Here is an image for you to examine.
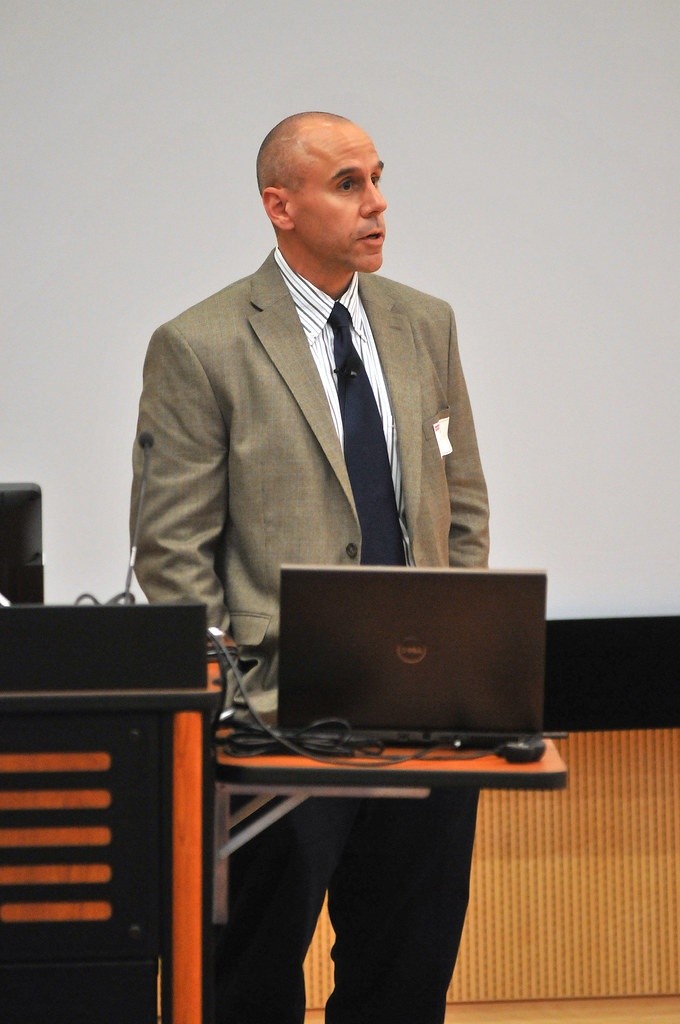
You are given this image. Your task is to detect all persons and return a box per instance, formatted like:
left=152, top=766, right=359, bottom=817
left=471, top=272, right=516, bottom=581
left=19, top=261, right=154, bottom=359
left=128, top=112, right=488, bottom=1024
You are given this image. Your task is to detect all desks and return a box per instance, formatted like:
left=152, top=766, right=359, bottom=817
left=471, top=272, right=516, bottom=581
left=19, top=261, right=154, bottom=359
left=211, top=715, right=568, bottom=926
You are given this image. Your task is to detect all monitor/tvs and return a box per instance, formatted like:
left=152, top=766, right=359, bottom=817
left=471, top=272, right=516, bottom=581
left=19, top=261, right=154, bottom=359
left=0, top=482, right=44, bottom=604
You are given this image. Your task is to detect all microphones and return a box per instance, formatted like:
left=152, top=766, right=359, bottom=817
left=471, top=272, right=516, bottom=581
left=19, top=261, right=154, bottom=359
left=125, top=432, right=154, bottom=606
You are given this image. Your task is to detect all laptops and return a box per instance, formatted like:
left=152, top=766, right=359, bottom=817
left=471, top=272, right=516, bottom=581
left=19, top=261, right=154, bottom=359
left=276, top=563, right=547, bottom=745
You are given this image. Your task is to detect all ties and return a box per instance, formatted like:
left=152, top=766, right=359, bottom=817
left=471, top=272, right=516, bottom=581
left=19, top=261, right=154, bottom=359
left=327, top=301, right=406, bottom=569
